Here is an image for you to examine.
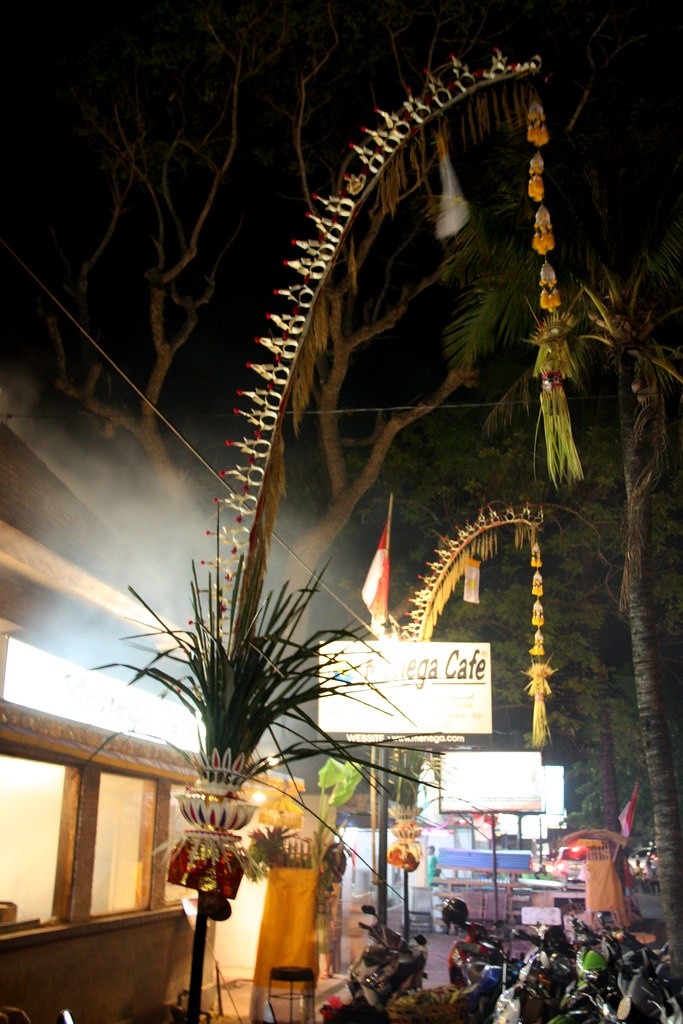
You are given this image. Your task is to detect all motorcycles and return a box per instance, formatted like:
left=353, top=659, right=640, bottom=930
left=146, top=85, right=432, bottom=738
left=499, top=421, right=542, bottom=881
left=344, top=897, right=683, bottom=1024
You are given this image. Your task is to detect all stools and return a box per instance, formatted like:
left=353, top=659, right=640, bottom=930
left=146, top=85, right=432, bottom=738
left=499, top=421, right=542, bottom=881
left=268, top=966, right=315, bottom=1000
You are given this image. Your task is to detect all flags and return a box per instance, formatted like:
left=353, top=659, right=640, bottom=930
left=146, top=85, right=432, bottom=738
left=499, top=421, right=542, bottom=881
left=618, top=783, right=637, bottom=848
left=361, top=523, right=389, bottom=618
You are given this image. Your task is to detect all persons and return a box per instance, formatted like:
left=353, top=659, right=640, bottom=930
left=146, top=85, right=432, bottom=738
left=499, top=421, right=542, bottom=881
left=315, top=886, right=334, bottom=980
left=427, top=846, right=437, bottom=888
left=521, top=866, right=553, bottom=880
left=624, top=853, right=661, bottom=897
